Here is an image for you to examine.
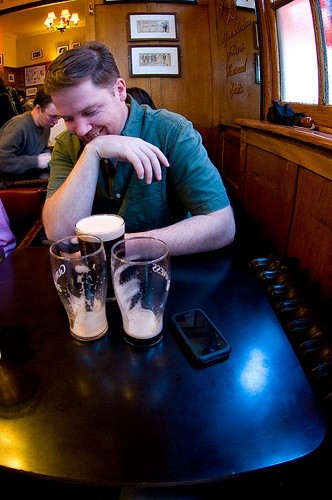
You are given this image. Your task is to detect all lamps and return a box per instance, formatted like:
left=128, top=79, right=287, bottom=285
left=44, top=7, right=80, bottom=35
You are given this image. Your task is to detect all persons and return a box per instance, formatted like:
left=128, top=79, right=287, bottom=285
left=0, top=199, right=17, bottom=258
left=43, top=42, right=236, bottom=262
left=126, top=86, right=157, bottom=110
left=0, top=78, right=60, bottom=173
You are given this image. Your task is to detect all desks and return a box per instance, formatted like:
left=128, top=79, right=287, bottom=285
left=0, top=238, right=327, bottom=488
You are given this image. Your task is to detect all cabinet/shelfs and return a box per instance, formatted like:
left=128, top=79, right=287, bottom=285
left=242, top=142, right=332, bottom=303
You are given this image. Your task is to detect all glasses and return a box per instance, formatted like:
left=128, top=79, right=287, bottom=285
left=41, top=106, right=61, bottom=121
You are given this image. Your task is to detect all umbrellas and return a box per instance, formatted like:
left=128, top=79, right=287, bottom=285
left=265, top=100, right=317, bottom=131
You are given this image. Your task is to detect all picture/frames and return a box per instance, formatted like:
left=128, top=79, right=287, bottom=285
left=128, top=44, right=181, bottom=78
left=26, top=87, right=37, bottom=97
left=24, top=64, right=46, bottom=87
left=31, top=49, right=42, bottom=59
left=56, top=45, right=68, bottom=54
left=125, top=11, right=180, bottom=42
left=8, top=74, right=15, bottom=82
left=0, top=54, right=3, bottom=65
left=72, top=42, right=81, bottom=48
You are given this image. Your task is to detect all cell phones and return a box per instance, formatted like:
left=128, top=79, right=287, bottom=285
left=42, top=239, right=80, bottom=253
left=170, top=308, right=232, bottom=366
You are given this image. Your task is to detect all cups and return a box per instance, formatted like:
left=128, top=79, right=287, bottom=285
left=49, top=233, right=109, bottom=342
left=75, top=213, right=129, bottom=302
left=110, top=237, right=171, bottom=349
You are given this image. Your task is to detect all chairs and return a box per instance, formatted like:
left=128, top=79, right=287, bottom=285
left=0, top=188, right=45, bottom=247
left=0, top=179, right=49, bottom=188
